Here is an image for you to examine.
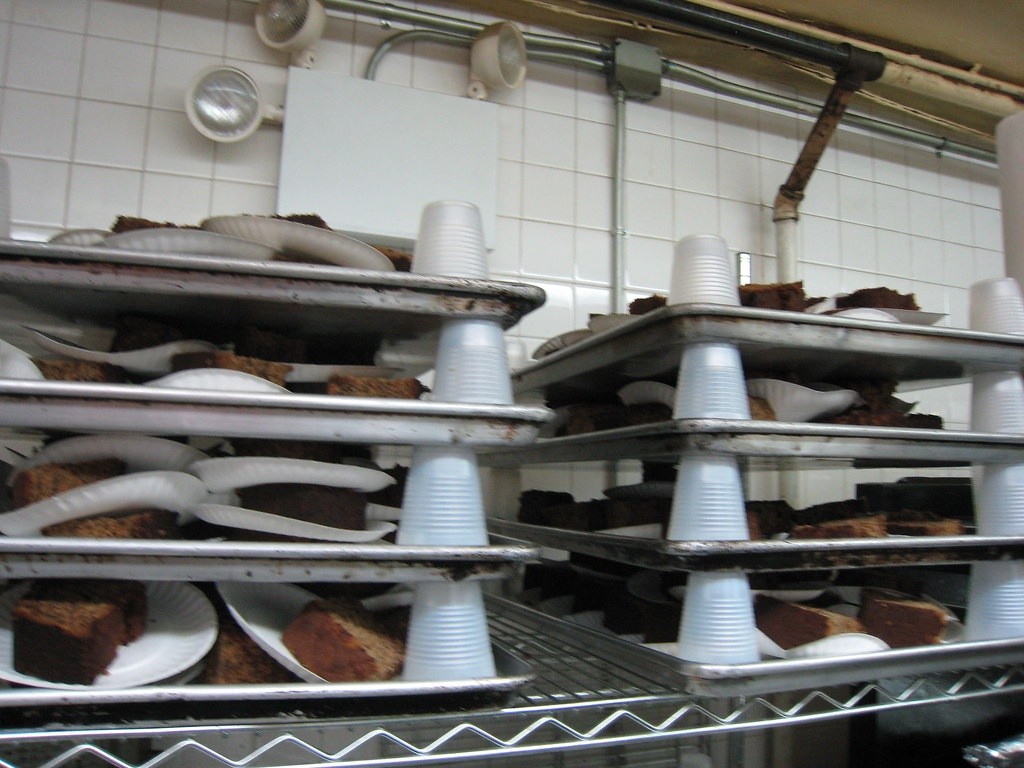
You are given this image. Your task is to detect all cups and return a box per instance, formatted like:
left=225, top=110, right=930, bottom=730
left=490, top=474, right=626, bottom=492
left=667, top=452, right=749, bottom=541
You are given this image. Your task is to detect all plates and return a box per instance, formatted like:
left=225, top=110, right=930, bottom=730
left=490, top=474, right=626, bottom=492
left=0, top=208, right=419, bottom=692
left=741, top=294, right=951, bottom=658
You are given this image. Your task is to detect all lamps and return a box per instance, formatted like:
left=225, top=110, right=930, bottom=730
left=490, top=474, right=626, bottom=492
left=252, top=0, right=326, bottom=68
left=467, top=21, right=529, bottom=98
left=183, top=64, right=286, bottom=144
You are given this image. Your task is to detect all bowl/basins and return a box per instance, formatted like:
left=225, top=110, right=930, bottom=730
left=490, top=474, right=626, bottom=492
left=965, top=562, right=1023, bottom=644
left=967, top=276, right=1023, bottom=337
left=678, top=571, right=761, bottom=665
left=971, top=370, right=1024, bottom=436
left=667, top=236, right=739, bottom=308
left=431, top=319, right=513, bottom=404
left=412, top=201, right=490, bottom=280
left=671, top=340, right=749, bottom=423
left=398, top=581, right=498, bottom=681
left=972, top=463, right=1023, bottom=537
left=394, top=447, right=489, bottom=549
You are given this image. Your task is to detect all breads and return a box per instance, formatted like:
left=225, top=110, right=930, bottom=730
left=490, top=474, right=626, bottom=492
left=6, top=211, right=424, bottom=680
left=514, top=280, right=967, bottom=654
